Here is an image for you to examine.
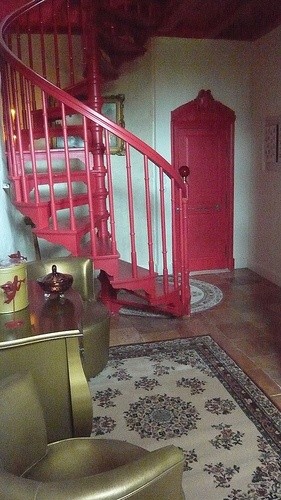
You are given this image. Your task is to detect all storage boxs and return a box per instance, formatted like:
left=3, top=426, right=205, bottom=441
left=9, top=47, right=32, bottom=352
left=0, top=258, right=30, bottom=313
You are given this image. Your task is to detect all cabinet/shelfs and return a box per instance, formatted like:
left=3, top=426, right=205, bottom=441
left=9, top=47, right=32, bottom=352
left=0, top=278, right=93, bottom=444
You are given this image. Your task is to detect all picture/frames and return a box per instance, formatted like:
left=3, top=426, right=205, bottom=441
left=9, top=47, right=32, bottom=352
left=262, top=116, right=281, bottom=172
left=47, top=93, right=126, bottom=156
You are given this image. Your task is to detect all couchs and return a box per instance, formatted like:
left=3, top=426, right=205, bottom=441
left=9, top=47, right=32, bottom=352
left=1, top=369, right=187, bottom=500
left=27, top=256, right=111, bottom=381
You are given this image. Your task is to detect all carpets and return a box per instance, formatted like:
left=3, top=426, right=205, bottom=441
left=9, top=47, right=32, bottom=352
left=114, top=274, right=224, bottom=318
left=87, top=334, right=281, bottom=500
